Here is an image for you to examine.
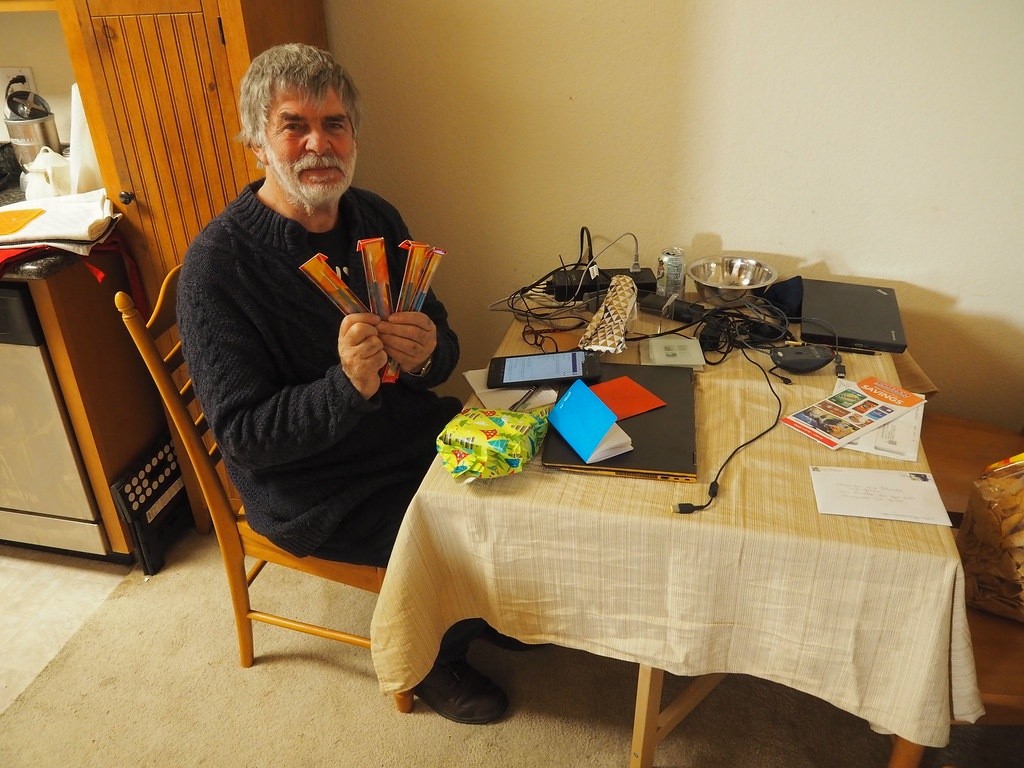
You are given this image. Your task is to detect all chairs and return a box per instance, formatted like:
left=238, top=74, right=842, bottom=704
left=116, top=265, right=415, bottom=713
left=885, top=610, right=1024, bottom=768
left=920, top=413, right=1024, bottom=523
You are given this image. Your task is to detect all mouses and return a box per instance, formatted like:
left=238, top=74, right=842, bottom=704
left=771, top=346, right=836, bottom=373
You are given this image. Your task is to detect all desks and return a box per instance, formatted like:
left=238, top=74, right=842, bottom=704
left=368, top=290, right=984, bottom=768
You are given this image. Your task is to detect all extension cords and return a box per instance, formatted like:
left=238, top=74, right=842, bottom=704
left=544, top=268, right=657, bottom=302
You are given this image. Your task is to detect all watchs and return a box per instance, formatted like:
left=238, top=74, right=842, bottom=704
left=408, top=355, right=434, bottom=377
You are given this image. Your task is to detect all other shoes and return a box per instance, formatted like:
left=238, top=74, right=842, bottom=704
left=475, top=622, right=541, bottom=651
left=412, top=648, right=509, bottom=724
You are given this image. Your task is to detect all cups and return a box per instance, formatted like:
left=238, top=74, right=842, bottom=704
left=5, top=114, right=59, bottom=171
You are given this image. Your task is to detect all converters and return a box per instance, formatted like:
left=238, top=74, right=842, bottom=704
left=583, top=288, right=704, bottom=323
left=699, top=316, right=729, bottom=350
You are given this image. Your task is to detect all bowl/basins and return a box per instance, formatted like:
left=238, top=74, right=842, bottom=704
left=686, top=256, right=777, bottom=305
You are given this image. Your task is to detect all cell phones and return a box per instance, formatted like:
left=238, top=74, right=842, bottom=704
left=487, top=351, right=601, bottom=388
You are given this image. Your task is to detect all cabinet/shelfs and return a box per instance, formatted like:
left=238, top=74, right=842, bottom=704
left=76, top=0, right=328, bottom=535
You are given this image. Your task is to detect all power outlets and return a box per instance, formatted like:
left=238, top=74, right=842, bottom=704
left=0, top=66, right=36, bottom=100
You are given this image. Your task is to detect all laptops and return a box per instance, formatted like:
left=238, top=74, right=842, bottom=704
left=542, top=363, right=697, bottom=482
left=801, top=279, right=907, bottom=353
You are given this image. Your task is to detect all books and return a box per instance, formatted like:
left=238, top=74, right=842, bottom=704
left=462, top=369, right=558, bottom=411
left=548, top=379, right=633, bottom=463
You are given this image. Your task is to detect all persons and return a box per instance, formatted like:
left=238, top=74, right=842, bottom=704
left=177, top=44, right=539, bottom=724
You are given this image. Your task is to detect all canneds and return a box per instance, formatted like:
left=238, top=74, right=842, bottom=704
left=656, top=247, right=686, bottom=299
